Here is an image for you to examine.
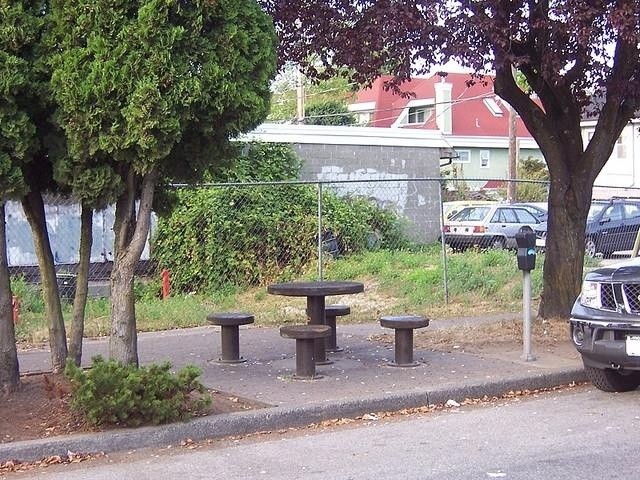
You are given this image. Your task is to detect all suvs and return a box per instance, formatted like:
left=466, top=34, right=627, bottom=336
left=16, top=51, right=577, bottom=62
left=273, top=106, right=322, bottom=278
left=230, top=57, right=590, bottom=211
left=568, top=255, right=640, bottom=391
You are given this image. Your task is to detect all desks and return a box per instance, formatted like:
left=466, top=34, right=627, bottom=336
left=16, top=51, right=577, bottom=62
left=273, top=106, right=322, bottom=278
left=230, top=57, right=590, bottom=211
left=268, top=281, right=364, bottom=365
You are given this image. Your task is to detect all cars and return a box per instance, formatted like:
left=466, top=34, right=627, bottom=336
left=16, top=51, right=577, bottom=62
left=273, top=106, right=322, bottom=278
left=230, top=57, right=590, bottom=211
left=534, top=196, right=640, bottom=258
left=442, top=201, right=548, bottom=249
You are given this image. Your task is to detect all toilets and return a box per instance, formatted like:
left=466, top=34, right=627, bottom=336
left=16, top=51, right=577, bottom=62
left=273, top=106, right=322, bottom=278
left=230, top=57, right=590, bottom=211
left=56, top=273, right=76, bottom=305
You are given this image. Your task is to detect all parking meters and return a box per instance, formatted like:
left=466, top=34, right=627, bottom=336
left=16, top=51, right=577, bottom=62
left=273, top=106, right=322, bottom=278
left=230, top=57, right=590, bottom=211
left=512, top=224, right=542, bottom=363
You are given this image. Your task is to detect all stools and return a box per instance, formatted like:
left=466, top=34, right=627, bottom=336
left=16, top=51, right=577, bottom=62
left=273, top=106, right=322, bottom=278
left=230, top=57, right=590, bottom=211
left=206, top=303, right=429, bottom=379
